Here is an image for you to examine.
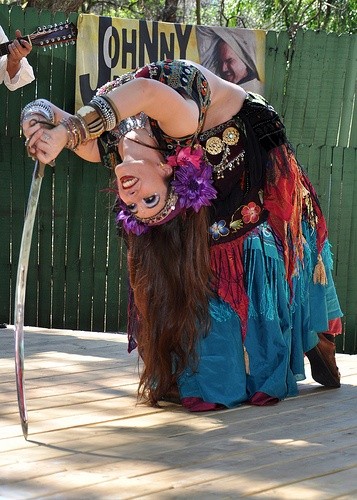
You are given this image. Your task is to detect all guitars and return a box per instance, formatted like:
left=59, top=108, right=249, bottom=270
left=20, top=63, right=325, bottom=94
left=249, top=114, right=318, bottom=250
left=1, top=17, right=79, bottom=60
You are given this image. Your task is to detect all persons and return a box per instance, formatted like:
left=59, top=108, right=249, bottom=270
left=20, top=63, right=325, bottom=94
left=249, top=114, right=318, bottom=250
left=21, top=59, right=343, bottom=412
left=0, top=26, right=35, bottom=91
left=196, top=26, right=263, bottom=96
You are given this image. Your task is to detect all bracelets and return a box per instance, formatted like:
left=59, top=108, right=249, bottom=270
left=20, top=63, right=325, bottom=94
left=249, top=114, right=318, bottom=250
left=59, top=95, right=122, bottom=151
left=20, top=100, right=55, bottom=125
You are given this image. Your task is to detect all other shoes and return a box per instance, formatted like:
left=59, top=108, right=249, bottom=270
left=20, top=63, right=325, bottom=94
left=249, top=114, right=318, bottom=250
left=304, top=332, right=341, bottom=388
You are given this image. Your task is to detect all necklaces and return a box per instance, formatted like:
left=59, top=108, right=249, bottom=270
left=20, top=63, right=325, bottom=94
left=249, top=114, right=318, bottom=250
left=121, top=134, right=158, bottom=150
